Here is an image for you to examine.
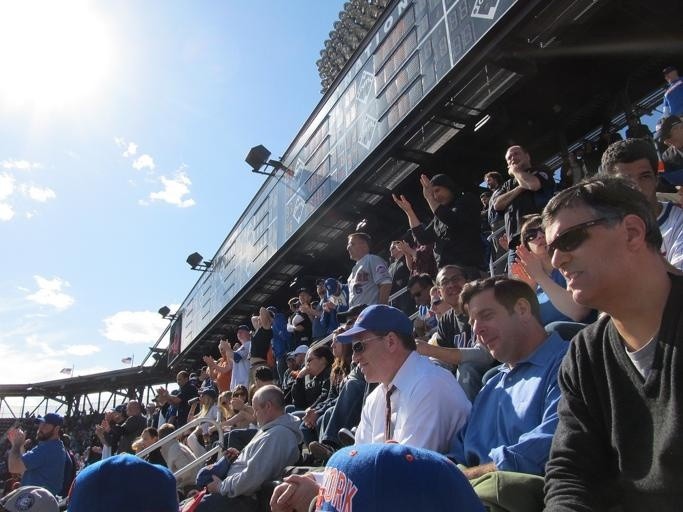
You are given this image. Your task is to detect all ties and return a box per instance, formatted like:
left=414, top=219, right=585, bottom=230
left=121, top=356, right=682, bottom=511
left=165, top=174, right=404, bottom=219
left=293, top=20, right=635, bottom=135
left=383, top=385, right=398, bottom=440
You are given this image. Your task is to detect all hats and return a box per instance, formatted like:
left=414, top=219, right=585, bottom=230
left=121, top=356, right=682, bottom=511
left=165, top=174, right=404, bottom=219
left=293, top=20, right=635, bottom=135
left=37, top=412, right=64, bottom=426
left=66, top=451, right=180, bottom=512
left=296, top=287, right=314, bottom=298
left=111, top=404, right=124, bottom=412
left=197, top=389, right=217, bottom=399
left=316, top=439, right=487, bottom=512
left=335, top=303, right=414, bottom=344
left=324, top=278, right=337, bottom=296
left=234, top=324, right=251, bottom=333
left=0, top=485, right=60, bottom=512
left=195, top=455, right=229, bottom=491
left=429, top=172, right=457, bottom=193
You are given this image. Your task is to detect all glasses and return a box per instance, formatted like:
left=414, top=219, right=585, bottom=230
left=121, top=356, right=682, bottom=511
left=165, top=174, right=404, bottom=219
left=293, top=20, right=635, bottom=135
left=351, top=334, right=383, bottom=355
left=232, top=392, right=242, bottom=397
left=523, top=226, right=545, bottom=242
left=290, top=344, right=310, bottom=356
left=548, top=215, right=622, bottom=259
left=414, top=287, right=426, bottom=298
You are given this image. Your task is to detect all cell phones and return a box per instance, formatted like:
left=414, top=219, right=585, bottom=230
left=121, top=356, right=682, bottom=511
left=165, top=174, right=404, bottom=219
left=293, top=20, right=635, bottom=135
left=219, top=442, right=232, bottom=455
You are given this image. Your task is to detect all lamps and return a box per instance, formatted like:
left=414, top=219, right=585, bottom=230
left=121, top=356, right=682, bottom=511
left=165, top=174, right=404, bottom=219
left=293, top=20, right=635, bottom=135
left=147, top=345, right=168, bottom=361
left=185, top=250, right=215, bottom=273
left=157, top=305, right=179, bottom=322
left=244, top=142, right=294, bottom=181
